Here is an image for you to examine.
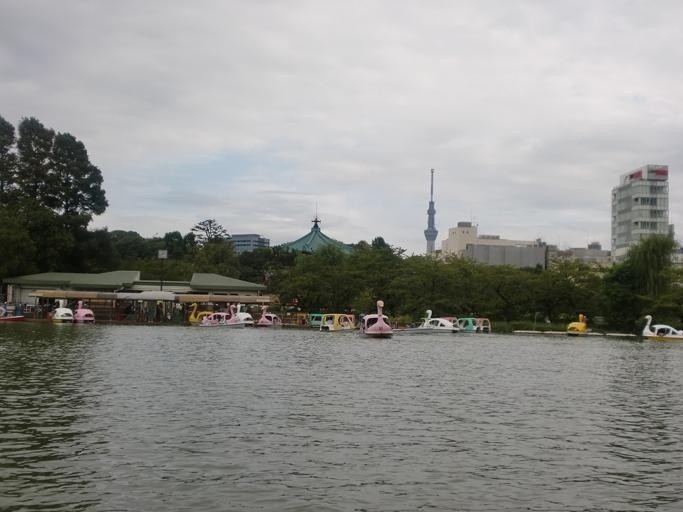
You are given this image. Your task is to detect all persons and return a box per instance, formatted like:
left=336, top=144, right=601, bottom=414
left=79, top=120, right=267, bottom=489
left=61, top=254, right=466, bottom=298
left=115, top=300, right=265, bottom=323
left=358, top=313, right=365, bottom=326
left=407, top=321, right=417, bottom=328
left=0, top=299, right=89, bottom=318
left=544, top=315, right=550, bottom=326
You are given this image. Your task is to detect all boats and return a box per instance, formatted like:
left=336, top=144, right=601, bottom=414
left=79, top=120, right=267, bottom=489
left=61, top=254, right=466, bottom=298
left=566, top=314, right=586, bottom=334
left=641, top=315, right=683, bottom=340
left=185, top=294, right=356, bottom=332
left=73, top=301, right=97, bottom=323
left=1, top=316, right=24, bottom=322
left=359, top=300, right=393, bottom=336
left=417, top=310, right=457, bottom=330
left=51, top=299, right=74, bottom=323
left=454, top=317, right=492, bottom=333
